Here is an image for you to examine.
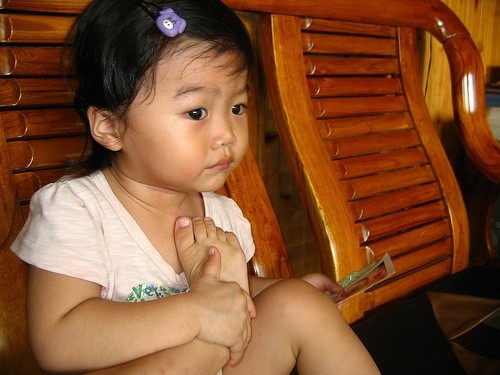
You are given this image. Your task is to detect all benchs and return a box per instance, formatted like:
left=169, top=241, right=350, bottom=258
left=0, top=0, right=500, bottom=375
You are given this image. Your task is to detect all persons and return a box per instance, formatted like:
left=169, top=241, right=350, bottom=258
left=10, top=1, right=381, bottom=375
